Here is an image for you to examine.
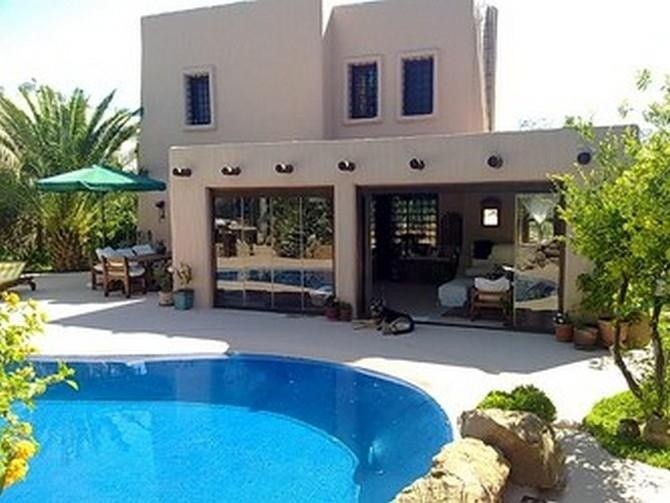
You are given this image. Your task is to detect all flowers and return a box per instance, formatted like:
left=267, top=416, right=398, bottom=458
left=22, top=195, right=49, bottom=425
left=551, top=310, right=569, bottom=325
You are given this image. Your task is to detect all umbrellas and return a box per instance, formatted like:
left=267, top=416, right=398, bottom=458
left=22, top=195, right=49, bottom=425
left=32, top=162, right=167, bottom=247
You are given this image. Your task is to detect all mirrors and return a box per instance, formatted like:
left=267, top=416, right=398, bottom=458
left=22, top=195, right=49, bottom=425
left=480, top=206, right=501, bottom=227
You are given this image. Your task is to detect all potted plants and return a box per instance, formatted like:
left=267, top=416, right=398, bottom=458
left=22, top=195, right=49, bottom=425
left=151, top=260, right=195, bottom=311
left=571, top=302, right=619, bottom=349
left=319, top=295, right=352, bottom=321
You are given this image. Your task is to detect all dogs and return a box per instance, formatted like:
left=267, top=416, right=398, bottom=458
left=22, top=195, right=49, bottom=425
left=368, top=295, right=415, bottom=335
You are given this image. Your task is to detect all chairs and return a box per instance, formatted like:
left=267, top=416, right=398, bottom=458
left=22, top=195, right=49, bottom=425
left=469, top=284, right=514, bottom=321
left=466, top=239, right=495, bottom=275
left=87, top=239, right=172, bottom=299
left=0, top=261, right=36, bottom=300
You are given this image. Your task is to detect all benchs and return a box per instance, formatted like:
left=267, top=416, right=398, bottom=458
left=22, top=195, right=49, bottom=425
left=436, top=275, right=474, bottom=317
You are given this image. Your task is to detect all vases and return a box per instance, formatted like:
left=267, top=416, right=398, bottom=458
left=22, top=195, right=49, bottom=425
left=555, top=324, right=573, bottom=342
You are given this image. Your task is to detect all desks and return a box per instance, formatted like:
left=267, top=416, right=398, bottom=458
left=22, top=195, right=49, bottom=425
left=399, top=255, right=456, bottom=282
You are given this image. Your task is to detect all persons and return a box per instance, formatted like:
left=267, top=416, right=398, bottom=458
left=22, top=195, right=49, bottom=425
left=521, top=247, right=551, bottom=270
